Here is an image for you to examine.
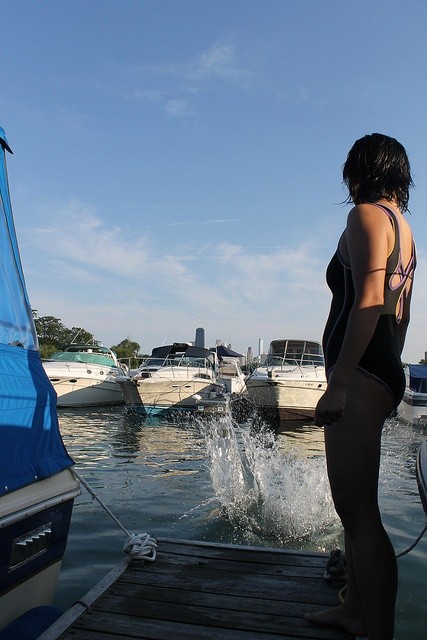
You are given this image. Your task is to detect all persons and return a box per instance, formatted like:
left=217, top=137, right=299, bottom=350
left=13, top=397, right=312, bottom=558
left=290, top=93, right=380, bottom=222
left=304, top=132, right=417, bottom=640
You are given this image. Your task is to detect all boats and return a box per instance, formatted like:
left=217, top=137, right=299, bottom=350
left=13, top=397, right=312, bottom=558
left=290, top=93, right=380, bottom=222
left=0, top=131, right=81, bottom=639
left=398, top=364, right=427, bottom=428
left=42, top=346, right=131, bottom=408
left=114, top=343, right=246, bottom=415
left=244, top=338, right=327, bottom=422
